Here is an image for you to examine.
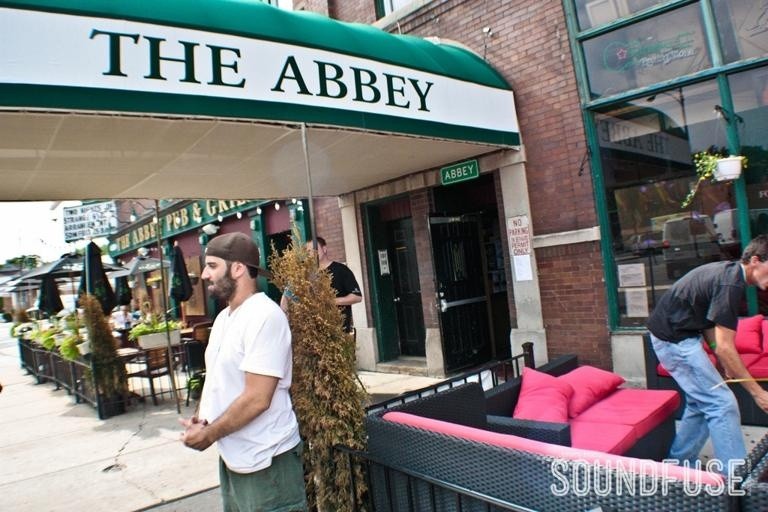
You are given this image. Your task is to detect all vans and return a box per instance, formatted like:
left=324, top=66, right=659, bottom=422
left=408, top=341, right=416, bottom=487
left=632, top=205, right=767, bottom=280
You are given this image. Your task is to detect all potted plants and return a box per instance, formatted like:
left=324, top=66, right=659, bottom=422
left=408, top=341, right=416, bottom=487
left=681, top=150, right=746, bottom=208
left=36, top=326, right=92, bottom=364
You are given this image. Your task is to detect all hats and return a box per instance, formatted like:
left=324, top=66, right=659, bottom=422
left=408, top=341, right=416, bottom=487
left=205, top=232, right=272, bottom=278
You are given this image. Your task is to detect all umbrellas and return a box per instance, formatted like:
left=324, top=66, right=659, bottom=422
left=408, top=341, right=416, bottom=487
left=0, top=236, right=195, bottom=323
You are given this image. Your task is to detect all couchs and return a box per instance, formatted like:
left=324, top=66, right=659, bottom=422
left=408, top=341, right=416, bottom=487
left=348, top=314, right=767, bottom=512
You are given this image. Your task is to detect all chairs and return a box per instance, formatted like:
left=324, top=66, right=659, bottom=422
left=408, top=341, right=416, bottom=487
left=114, top=320, right=212, bottom=408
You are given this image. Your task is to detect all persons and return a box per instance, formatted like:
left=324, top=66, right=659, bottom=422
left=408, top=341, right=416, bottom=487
left=128, top=301, right=155, bottom=328
left=109, top=303, right=132, bottom=338
left=644, top=233, right=768, bottom=480
left=278, top=261, right=309, bottom=314
left=177, top=231, right=311, bottom=512
left=304, top=235, right=364, bottom=338
